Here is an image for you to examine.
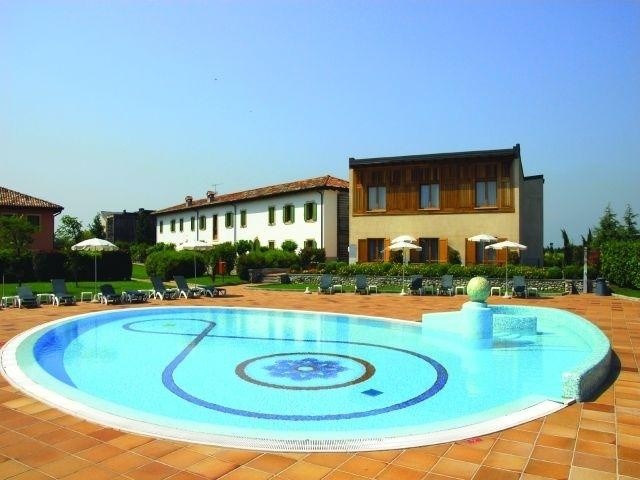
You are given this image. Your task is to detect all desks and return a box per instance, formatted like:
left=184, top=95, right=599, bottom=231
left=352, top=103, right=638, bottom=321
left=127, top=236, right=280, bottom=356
left=1, top=296, right=15, bottom=308
left=368, top=285, right=378, bottom=293
left=490, top=286, right=503, bottom=296
left=455, top=286, right=466, bottom=295
left=81, top=291, right=92, bottom=303
left=527, top=287, right=538, bottom=297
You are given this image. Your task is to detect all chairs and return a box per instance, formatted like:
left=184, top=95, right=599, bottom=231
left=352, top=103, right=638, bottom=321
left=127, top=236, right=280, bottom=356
left=16, top=279, right=74, bottom=307
left=512, top=275, right=528, bottom=298
left=99, top=272, right=226, bottom=305
left=317, top=273, right=370, bottom=295
left=407, top=274, right=455, bottom=296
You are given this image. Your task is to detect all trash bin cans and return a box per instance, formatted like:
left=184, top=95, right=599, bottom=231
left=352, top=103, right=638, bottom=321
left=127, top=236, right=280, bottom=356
left=248, top=272, right=255, bottom=282
left=596, top=278, right=606, bottom=295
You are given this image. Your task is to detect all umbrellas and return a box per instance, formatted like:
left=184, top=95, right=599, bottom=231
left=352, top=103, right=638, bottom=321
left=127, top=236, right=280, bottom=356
left=389, top=242, right=423, bottom=289
left=391, top=235, right=417, bottom=262
left=468, top=233, right=498, bottom=264
left=484, top=241, right=527, bottom=291
left=71, top=238, right=119, bottom=295
